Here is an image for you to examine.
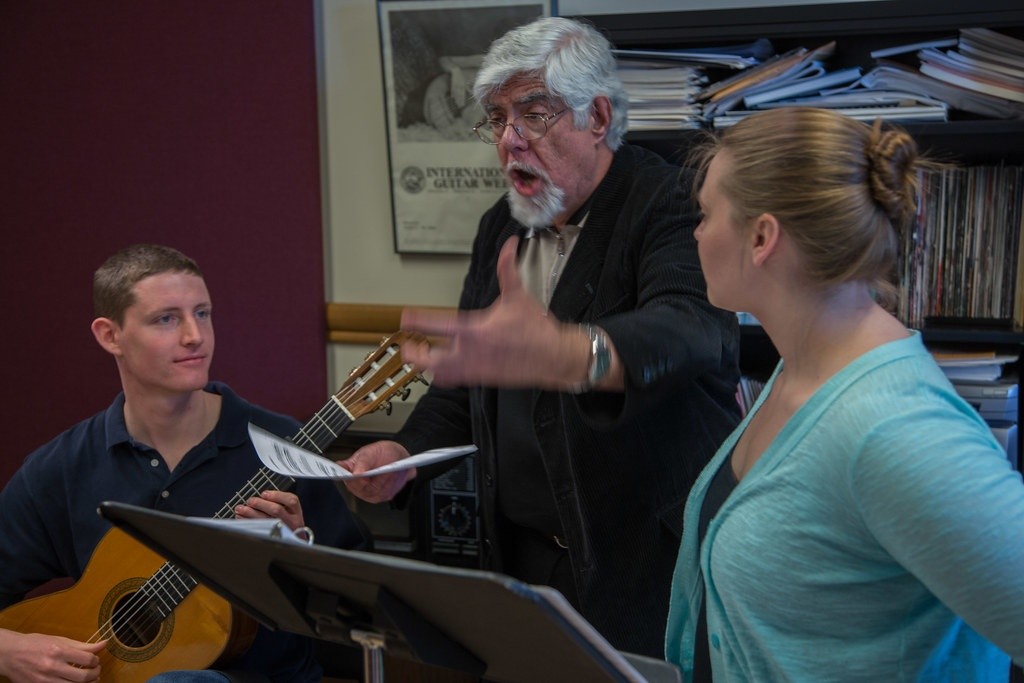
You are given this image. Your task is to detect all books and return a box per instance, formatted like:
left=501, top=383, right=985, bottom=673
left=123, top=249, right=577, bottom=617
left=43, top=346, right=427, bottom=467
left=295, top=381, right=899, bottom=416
left=608, top=25, right=1024, bottom=131
left=892, top=159, right=1024, bottom=331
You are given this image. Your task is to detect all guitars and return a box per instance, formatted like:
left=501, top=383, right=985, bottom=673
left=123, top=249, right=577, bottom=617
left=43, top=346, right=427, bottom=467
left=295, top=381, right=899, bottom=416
left=0, top=326, right=435, bottom=683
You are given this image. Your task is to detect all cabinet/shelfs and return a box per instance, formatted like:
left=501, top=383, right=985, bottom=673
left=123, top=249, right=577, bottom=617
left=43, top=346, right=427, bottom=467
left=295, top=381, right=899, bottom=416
left=552, top=0, right=1024, bottom=477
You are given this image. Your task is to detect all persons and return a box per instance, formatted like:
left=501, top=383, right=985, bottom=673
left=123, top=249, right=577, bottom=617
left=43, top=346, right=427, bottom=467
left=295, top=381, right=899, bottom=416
left=336, top=14, right=745, bottom=658
left=662, top=106, right=1024, bottom=683
left=0, top=241, right=373, bottom=683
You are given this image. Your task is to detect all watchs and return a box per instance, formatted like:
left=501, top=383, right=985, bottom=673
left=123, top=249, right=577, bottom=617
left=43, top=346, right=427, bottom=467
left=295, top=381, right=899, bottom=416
left=570, top=325, right=612, bottom=395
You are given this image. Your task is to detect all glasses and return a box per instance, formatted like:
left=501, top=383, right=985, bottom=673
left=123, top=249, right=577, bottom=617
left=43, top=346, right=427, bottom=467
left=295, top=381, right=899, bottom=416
left=473, top=104, right=572, bottom=145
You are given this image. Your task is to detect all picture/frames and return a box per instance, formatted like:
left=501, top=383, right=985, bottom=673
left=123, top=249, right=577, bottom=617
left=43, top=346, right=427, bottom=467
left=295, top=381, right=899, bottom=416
left=376, top=0, right=556, bottom=255
